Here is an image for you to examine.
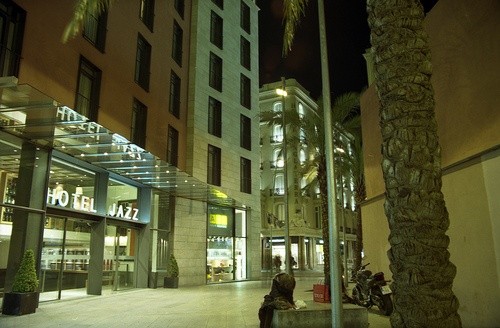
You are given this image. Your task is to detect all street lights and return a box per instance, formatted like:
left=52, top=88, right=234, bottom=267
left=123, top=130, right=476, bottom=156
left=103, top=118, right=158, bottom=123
left=275, top=75, right=293, bottom=277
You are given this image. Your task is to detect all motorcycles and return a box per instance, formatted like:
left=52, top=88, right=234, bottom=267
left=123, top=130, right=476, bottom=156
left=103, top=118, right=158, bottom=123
left=351, top=262, right=395, bottom=316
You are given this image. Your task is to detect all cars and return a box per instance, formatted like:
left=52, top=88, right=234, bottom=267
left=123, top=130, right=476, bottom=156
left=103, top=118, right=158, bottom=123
left=42, top=247, right=90, bottom=267
